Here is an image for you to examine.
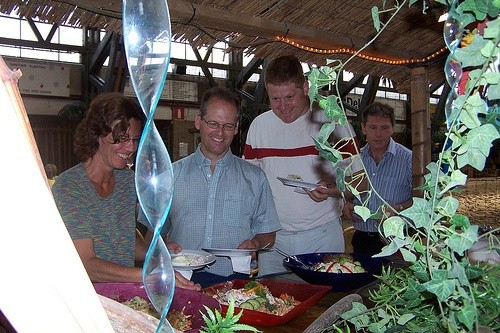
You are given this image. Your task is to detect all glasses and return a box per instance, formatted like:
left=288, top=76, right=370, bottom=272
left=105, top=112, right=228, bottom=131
left=108, top=135, right=141, bottom=144
left=202, top=117, right=237, bottom=131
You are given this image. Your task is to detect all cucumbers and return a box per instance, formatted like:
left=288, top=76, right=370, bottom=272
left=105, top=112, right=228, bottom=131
left=353, top=261, right=364, bottom=273
left=239, top=298, right=268, bottom=310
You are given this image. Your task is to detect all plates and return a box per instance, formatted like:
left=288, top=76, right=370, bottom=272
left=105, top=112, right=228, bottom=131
left=201, top=248, right=258, bottom=256
left=276, top=176, right=322, bottom=190
left=195, top=278, right=332, bottom=327
left=168, top=249, right=216, bottom=271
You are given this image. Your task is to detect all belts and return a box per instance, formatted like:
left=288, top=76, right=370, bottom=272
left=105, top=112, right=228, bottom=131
left=357, top=230, right=385, bottom=236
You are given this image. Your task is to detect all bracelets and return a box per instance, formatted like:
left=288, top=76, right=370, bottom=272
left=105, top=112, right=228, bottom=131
left=399, top=204, right=403, bottom=210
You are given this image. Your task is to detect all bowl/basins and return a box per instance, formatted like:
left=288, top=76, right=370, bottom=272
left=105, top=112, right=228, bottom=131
left=282, top=252, right=389, bottom=292
left=92, top=282, right=222, bottom=333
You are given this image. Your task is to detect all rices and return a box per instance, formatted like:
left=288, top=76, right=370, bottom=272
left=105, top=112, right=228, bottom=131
left=171, top=255, right=204, bottom=266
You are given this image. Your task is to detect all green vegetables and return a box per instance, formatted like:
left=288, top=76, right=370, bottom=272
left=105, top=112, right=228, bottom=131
left=331, top=254, right=350, bottom=265
left=244, top=282, right=267, bottom=298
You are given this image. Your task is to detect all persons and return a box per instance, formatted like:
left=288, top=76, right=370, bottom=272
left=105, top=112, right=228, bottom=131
left=51, top=91, right=202, bottom=292
left=137, top=86, right=282, bottom=289
left=343, top=102, right=413, bottom=252
left=241, top=54, right=366, bottom=277
